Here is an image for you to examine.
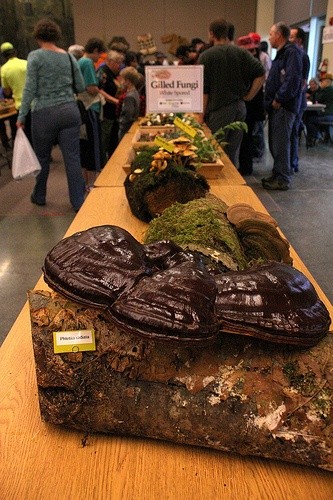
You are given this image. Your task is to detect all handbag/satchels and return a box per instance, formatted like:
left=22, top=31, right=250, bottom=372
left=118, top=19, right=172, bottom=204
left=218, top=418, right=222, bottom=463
left=77, top=99, right=88, bottom=125
left=11, top=126, right=42, bottom=182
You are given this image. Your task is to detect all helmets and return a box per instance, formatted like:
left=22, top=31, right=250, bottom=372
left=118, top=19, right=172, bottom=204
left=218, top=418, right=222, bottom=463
left=0, top=42, right=14, bottom=52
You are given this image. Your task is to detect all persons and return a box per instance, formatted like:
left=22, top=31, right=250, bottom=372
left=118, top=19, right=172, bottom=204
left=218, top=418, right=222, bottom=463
left=175, top=17, right=310, bottom=190
left=0, top=36, right=172, bottom=192
left=302, top=74, right=333, bottom=148
left=16, top=17, right=87, bottom=211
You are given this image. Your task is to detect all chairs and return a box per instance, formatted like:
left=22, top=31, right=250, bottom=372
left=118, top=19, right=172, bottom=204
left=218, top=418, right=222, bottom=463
left=319, top=118, right=333, bottom=144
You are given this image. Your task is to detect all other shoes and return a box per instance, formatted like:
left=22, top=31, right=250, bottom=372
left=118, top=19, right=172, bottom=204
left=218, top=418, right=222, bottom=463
left=260, top=171, right=290, bottom=191
left=31, top=193, right=46, bottom=206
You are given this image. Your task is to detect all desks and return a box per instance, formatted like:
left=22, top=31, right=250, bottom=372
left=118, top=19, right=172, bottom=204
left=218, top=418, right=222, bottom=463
left=94, top=125, right=249, bottom=184
left=0, top=186, right=333, bottom=499
left=301, top=101, right=327, bottom=147
left=0, top=110, right=17, bottom=176
left=128, top=115, right=212, bottom=132
